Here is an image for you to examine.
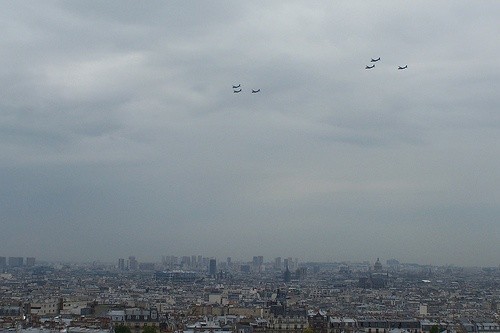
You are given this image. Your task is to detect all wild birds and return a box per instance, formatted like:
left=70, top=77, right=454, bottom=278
left=365, top=64, right=375, bottom=69
left=233, top=89, right=242, bottom=93
left=398, top=64, right=408, bottom=69
left=371, top=58, right=380, bottom=62
left=251, top=89, right=260, bottom=93
left=232, top=84, right=240, bottom=88
